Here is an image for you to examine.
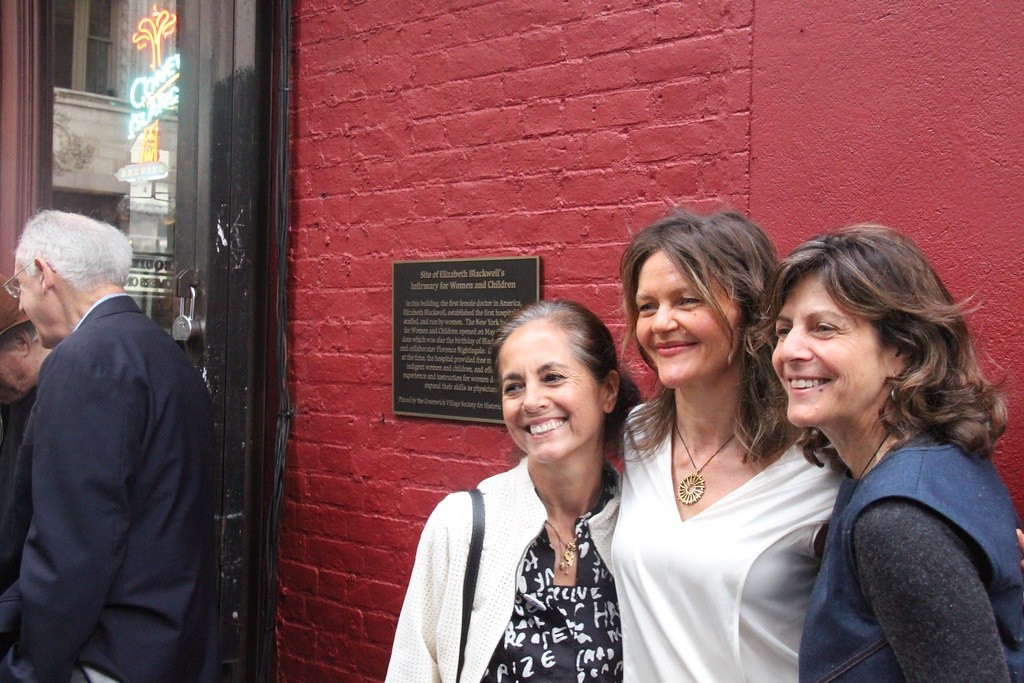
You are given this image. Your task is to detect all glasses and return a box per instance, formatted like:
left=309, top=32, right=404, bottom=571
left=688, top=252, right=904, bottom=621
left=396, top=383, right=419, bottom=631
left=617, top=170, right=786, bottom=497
left=2, top=258, right=56, bottom=298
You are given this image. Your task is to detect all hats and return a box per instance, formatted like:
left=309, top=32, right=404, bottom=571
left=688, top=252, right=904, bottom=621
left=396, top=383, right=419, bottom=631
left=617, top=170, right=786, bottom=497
left=0, top=273, right=31, bottom=334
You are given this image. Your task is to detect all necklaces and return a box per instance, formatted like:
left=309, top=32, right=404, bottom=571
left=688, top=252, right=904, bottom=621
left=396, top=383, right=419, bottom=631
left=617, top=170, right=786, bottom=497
left=547, top=521, right=577, bottom=575
left=846, top=428, right=891, bottom=500
left=676, top=417, right=748, bottom=504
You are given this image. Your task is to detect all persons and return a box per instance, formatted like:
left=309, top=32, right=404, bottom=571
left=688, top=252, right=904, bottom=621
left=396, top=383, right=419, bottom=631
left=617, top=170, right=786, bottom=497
left=384, top=301, right=642, bottom=683
left=612, top=212, right=1024, bottom=683
left=749, top=222, right=1024, bottom=683
left=0, top=210, right=217, bottom=683
